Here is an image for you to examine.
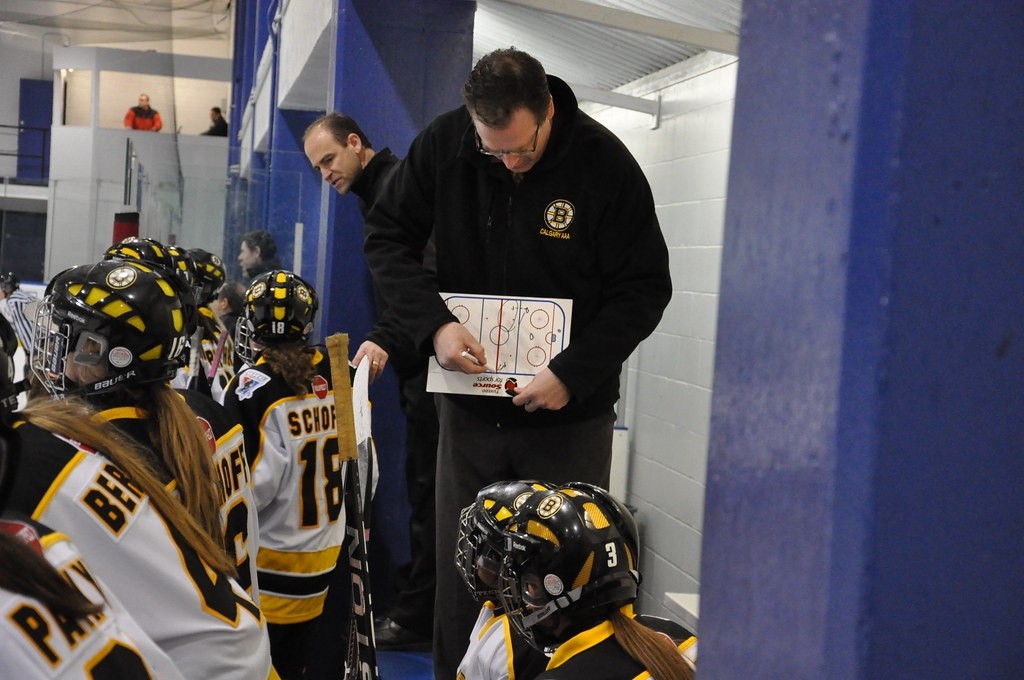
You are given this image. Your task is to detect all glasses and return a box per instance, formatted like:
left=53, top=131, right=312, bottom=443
left=471, top=124, right=540, bottom=158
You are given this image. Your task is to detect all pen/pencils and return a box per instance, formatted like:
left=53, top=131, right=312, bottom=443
left=461, top=351, right=496, bottom=372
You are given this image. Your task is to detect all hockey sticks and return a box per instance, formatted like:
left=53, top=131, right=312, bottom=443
left=322, top=327, right=381, bottom=680
left=205, top=330, right=230, bottom=394
left=186, top=325, right=205, bottom=390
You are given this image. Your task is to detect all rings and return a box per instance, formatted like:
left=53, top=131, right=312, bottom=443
left=373, top=361, right=378, bottom=365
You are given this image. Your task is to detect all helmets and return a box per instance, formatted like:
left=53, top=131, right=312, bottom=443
left=0, top=272, right=20, bottom=294
left=499, top=480, right=640, bottom=657
left=27, top=235, right=226, bottom=400
left=233, top=270, right=313, bottom=368
left=455, top=478, right=560, bottom=599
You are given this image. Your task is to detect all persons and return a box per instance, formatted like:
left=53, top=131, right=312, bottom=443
left=201, top=107, right=227, bottom=136
left=304, top=111, right=440, bottom=652
left=123, top=95, right=162, bottom=132
left=0, top=230, right=380, bottom=680
left=358, top=48, right=673, bottom=680
left=456, top=479, right=698, bottom=680
